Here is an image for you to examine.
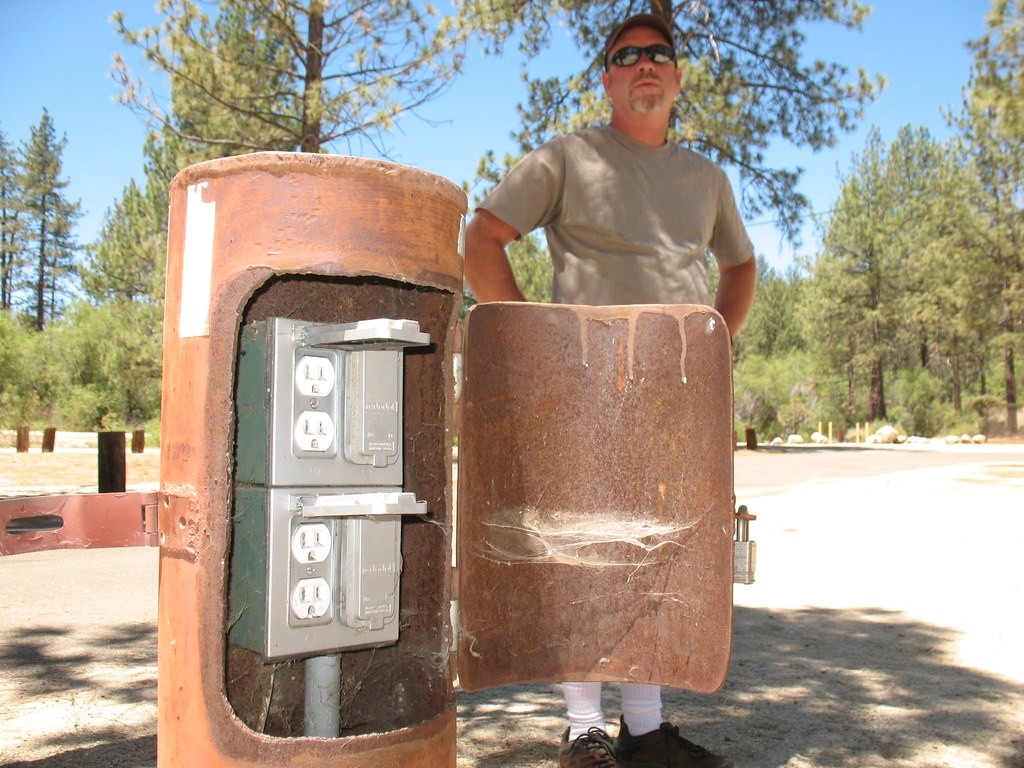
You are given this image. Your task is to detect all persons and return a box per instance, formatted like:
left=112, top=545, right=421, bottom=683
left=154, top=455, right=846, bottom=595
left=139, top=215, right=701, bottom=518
left=462, top=12, right=758, bottom=768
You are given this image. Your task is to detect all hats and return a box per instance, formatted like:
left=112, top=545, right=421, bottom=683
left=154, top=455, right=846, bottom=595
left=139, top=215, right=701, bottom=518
left=603, top=14, right=678, bottom=71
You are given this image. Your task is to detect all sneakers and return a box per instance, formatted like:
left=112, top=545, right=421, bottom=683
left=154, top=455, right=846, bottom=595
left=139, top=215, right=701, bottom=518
left=616, top=713, right=726, bottom=768
left=558, top=726, right=619, bottom=768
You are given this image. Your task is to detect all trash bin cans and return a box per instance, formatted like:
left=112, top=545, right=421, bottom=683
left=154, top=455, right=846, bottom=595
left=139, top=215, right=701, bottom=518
left=99, top=432, right=127, bottom=492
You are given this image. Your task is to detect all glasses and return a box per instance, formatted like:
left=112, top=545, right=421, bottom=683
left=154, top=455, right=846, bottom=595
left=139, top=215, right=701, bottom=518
left=605, top=43, right=675, bottom=71
